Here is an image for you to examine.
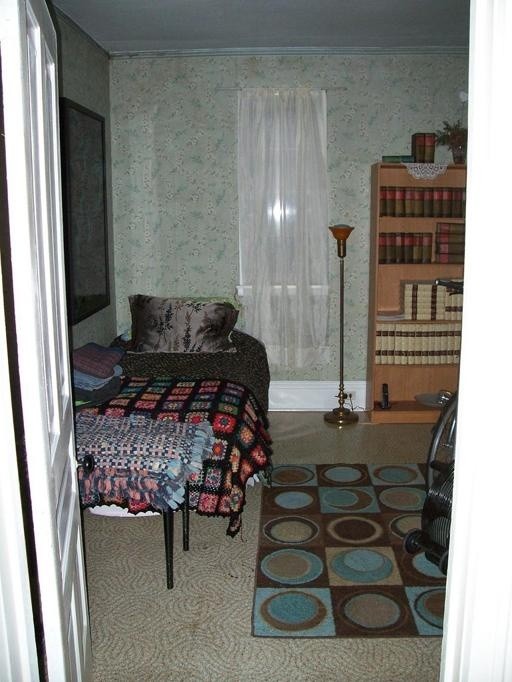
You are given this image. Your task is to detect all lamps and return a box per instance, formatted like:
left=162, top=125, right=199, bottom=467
left=323, top=224, right=358, bottom=426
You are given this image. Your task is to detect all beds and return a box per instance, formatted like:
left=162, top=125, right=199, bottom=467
left=73, top=327, right=272, bottom=541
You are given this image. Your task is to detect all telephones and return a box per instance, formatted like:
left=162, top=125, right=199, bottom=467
left=380, top=384, right=391, bottom=409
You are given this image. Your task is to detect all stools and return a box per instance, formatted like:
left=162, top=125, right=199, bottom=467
left=71, top=414, right=212, bottom=590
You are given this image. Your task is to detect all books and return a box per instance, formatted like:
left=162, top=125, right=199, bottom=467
left=374, top=186, right=466, bottom=366
left=381, top=383, right=389, bottom=409
left=381, top=133, right=436, bottom=164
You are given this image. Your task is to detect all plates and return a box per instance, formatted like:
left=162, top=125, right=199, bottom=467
left=415, top=394, right=444, bottom=407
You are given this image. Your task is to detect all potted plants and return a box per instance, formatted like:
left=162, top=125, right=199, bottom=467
left=434, top=119, right=468, bottom=163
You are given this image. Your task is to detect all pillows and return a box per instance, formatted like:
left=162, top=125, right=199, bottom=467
left=127, top=294, right=238, bottom=355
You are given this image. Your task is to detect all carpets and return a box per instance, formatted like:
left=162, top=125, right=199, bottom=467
left=250, top=462, right=450, bottom=637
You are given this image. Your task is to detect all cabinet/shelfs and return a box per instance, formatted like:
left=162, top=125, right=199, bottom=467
left=366, top=164, right=467, bottom=425
